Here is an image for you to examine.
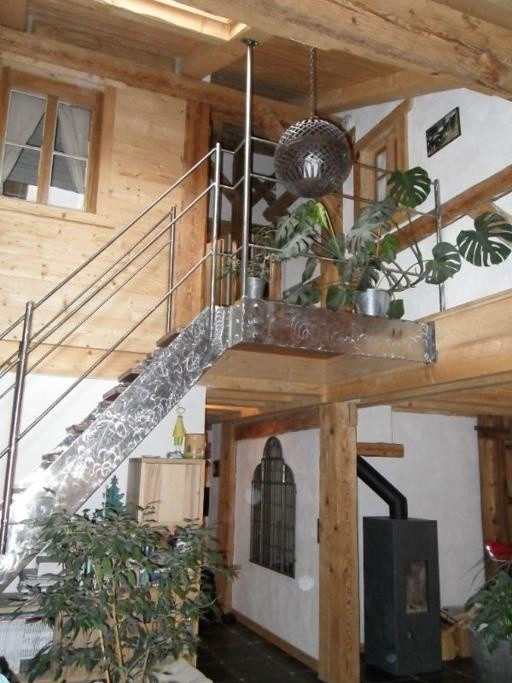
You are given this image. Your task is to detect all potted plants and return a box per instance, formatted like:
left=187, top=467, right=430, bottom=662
left=458, top=549, right=511, bottom=682
left=273, top=168, right=512, bottom=318
left=211, top=222, right=274, bottom=299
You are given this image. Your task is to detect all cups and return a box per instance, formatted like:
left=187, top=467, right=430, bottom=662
left=184, top=434, right=206, bottom=460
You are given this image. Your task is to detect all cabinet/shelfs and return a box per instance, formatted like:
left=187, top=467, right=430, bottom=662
left=128, top=458, right=206, bottom=669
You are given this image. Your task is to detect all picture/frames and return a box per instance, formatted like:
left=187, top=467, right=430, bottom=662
left=426, top=107, right=460, bottom=157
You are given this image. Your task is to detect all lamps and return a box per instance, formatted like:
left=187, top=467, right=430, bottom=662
left=272, top=47, right=353, bottom=199
